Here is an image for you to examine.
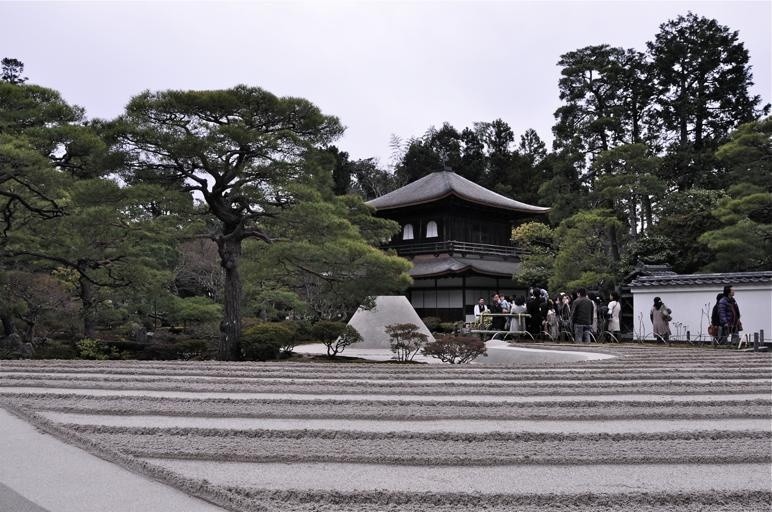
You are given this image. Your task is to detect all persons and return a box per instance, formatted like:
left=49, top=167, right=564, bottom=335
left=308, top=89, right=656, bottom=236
left=650, top=297, right=672, bottom=344
left=474, top=283, right=621, bottom=343
left=711, top=285, right=740, bottom=345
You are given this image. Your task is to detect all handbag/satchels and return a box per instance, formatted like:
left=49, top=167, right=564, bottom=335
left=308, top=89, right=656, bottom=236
left=707, top=325, right=720, bottom=337
left=660, top=305, right=672, bottom=321
